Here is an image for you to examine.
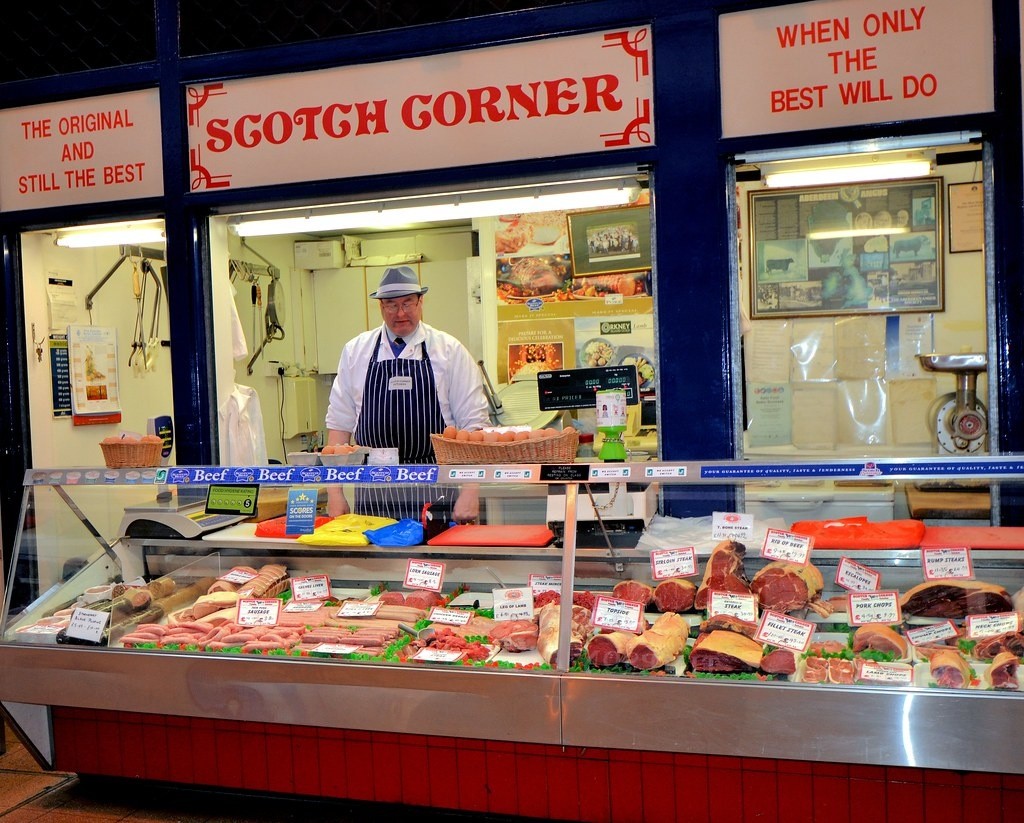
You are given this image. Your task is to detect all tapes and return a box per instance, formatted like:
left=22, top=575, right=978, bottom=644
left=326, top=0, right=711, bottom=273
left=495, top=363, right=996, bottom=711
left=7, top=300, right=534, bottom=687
left=422, top=503, right=432, bottom=531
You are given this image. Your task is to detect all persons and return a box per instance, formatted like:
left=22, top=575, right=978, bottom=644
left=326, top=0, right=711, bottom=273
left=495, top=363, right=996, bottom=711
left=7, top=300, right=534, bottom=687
left=324, top=266, right=492, bottom=524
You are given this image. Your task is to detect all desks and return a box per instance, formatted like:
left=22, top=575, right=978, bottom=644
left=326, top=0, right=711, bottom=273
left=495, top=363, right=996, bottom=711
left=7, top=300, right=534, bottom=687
left=903, top=483, right=993, bottom=527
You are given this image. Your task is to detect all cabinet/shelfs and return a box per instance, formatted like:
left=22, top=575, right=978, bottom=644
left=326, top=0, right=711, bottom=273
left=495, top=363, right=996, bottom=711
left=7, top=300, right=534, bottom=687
left=313, top=255, right=483, bottom=372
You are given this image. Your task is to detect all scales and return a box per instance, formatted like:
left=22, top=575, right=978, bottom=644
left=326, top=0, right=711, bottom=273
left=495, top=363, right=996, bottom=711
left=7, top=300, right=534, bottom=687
left=537, top=364, right=659, bottom=537
left=116, top=496, right=259, bottom=540
left=912, top=352, right=994, bottom=492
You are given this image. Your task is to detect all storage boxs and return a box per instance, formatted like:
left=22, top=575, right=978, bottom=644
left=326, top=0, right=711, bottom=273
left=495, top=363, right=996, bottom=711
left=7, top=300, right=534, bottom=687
left=286, top=453, right=320, bottom=466
left=416, top=231, right=479, bottom=259
left=320, top=446, right=370, bottom=468
left=360, top=236, right=415, bottom=255
left=294, top=239, right=345, bottom=271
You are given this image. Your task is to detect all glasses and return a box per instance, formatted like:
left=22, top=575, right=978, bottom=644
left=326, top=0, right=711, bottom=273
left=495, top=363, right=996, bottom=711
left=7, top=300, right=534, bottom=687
left=381, top=297, right=419, bottom=312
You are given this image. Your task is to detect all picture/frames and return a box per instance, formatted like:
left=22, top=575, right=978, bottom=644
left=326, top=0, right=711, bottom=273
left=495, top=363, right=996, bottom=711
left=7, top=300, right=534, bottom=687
left=564, top=204, right=656, bottom=276
left=746, top=173, right=948, bottom=321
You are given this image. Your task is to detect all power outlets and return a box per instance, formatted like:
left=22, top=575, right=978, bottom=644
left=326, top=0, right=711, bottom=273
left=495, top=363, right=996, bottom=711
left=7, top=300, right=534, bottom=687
left=265, top=362, right=285, bottom=378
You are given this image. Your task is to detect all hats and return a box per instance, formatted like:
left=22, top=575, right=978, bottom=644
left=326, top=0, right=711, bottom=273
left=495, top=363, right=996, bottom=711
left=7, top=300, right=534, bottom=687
left=369, top=266, right=428, bottom=298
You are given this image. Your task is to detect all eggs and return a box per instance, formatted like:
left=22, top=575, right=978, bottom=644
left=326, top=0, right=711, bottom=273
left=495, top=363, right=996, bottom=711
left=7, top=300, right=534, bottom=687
left=321, top=445, right=361, bottom=454
left=442, top=426, right=574, bottom=442
left=103, top=434, right=161, bottom=442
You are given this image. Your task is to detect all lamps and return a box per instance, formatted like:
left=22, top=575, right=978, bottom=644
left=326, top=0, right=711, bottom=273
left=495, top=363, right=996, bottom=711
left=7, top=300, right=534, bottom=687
left=761, top=147, right=935, bottom=188
left=229, top=175, right=644, bottom=241
left=53, top=220, right=167, bottom=251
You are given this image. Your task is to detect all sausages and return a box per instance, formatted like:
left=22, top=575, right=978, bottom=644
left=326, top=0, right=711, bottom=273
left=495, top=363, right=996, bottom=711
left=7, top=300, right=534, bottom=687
left=56, top=566, right=425, bottom=660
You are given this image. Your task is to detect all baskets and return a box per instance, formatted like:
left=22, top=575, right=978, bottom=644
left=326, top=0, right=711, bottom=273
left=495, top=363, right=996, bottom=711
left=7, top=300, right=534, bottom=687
left=99, top=441, right=165, bottom=468
left=431, top=430, right=579, bottom=463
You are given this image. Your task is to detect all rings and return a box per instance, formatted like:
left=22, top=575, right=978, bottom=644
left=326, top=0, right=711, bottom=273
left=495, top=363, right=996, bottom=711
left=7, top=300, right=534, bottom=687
left=472, top=520, right=475, bottom=522
